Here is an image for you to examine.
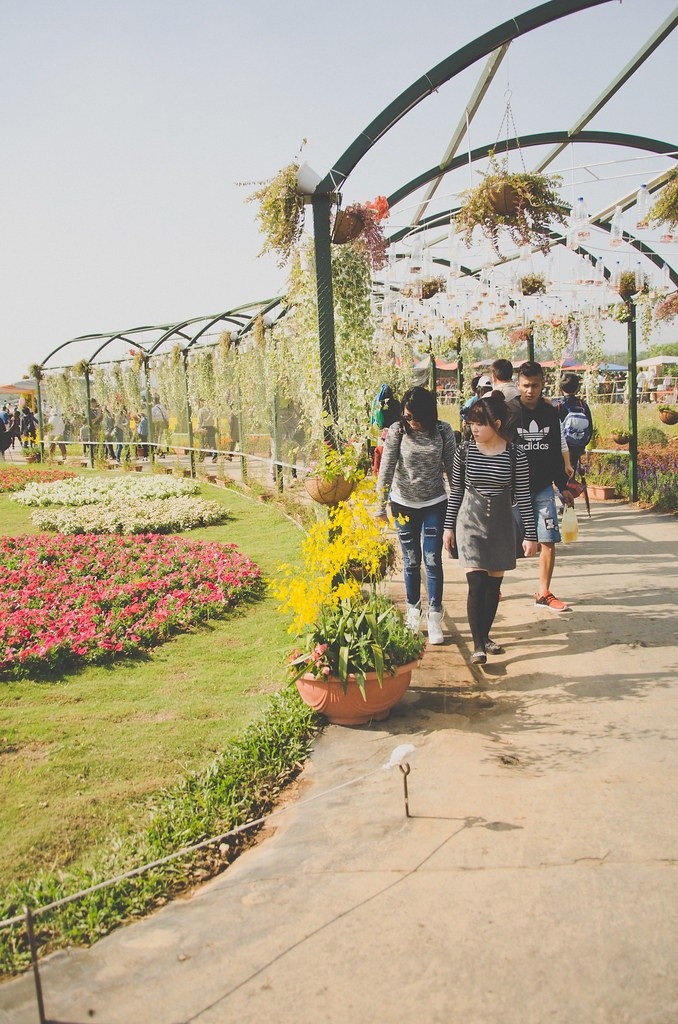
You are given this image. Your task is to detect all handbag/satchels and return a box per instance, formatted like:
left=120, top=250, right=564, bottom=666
left=561, top=504, right=579, bottom=544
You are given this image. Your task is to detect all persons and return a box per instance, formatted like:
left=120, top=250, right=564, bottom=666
left=482, top=359, right=521, bottom=402
left=73, top=392, right=169, bottom=463
left=372, top=386, right=457, bottom=645
left=196, top=397, right=218, bottom=464
left=453, top=377, right=493, bottom=446
left=224, top=401, right=239, bottom=462
left=443, top=390, right=538, bottom=663
left=503, top=361, right=574, bottom=611
left=267, top=399, right=311, bottom=488
left=0, top=397, right=73, bottom=461
left=414, top=367, right=673, bottom=405
left=551, top=371, right=593, bottom=515
left=356, top=385, right=402, bottom=480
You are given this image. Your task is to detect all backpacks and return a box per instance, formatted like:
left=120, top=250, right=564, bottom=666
left=555, top=399, right=591, bottom=447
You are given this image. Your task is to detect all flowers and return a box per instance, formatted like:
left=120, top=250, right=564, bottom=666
left=0, top=151, right=572, bottom=702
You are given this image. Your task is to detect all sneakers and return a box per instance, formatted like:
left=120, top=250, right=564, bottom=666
left=426, top=606, right=444, bottom=645
left=471, top=646, right=487, bottom=664
left=483, top=639, right=501, bottom=654
left=405, top=598, right=423, bottom=633
left=534, top=592, right=568, bottom=612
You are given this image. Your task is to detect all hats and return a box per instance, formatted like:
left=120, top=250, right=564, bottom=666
left=378, top=384, right=393, bottom=401
left=476, top=376, right=492, bottom=388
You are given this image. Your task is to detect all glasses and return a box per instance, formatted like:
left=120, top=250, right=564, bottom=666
left=402, top=407, right=420, bottom=422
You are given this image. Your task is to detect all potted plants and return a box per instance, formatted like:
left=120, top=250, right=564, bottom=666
left=580, top=405, right=678, bottom=499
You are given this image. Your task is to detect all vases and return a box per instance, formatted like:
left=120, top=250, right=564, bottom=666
left=174, top=448, right=187, bottom=455
left=352, top=553, right=392, bottom=581
left=421, top=283, right=437, bottom=299
left=133, top=465, right=143, bottom=470
left=80, top=463, right=88, bottom=468
left=103, top=464, right=115, bottom=470
left=161, top=469, right=171, bottom=474
left=137, top=447, right=145, bottom=457
left=333, top=212, right=363, bottom=243
left=258, top=493, right=274, bottom=503
left=305, top=476, right=355, bottom=506
left=53, top=460, right=61, bottom=466
left=182, top=470, right=195, bottom=477
left=290, top=661, right=419, bottom=725
left=482, top=183, right=524, bottom=215
left=295, top=513, right=305, bottom=525
left=24, top=456, right=36, bottom=463
left=294, top=160, right=321, bottom=194
left=205, top=476, right=234, bottom=487
left=277, top=500, right=289, bottom=512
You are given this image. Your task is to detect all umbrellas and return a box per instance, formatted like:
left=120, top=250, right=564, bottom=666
left=0, top=374, right=96, bottom=410
left=394, top=356, right=678, bottom=370
